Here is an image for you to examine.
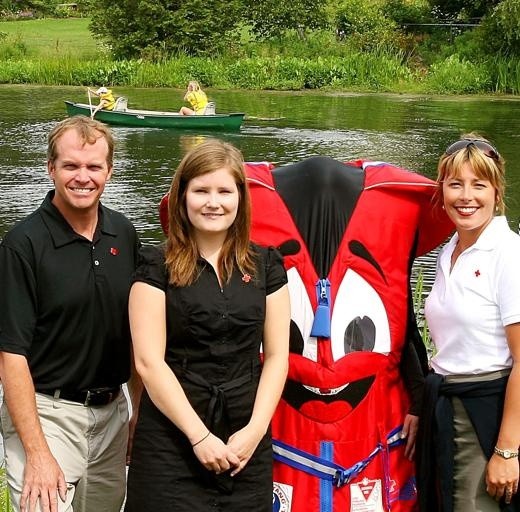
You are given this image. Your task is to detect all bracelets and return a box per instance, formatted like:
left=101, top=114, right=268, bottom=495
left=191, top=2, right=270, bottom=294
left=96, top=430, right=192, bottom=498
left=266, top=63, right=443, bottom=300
left=192, top=431, right=211, bottom=448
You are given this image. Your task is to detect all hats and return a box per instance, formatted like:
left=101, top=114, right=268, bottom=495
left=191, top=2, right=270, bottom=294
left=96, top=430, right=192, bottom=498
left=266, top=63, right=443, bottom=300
left=96, top=87, right=108, bottom=93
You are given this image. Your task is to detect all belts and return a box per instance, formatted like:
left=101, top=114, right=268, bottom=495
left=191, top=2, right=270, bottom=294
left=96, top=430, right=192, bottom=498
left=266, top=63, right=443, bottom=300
left=33, top=388, right=121, bottom=408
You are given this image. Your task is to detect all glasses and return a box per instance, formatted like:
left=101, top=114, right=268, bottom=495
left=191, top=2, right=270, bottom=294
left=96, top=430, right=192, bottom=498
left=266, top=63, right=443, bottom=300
left=446, top=139, right=499, bottom=160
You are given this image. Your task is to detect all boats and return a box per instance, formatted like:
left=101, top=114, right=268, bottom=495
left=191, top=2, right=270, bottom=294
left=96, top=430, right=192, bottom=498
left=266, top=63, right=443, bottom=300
left=63, top=99, right=244, bottom=133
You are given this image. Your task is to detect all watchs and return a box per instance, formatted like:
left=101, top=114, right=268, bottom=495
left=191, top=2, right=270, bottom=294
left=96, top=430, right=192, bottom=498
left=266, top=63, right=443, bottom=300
left=494, top=446, right=519, bottom=459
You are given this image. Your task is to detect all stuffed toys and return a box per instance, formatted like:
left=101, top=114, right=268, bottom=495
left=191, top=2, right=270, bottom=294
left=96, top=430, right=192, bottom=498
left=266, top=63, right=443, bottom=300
left=158, top=155, right=458, bottom=512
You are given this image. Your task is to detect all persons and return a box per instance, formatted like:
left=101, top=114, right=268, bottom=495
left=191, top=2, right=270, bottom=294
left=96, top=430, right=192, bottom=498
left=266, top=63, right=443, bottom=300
left=124, top=140, right=292, bottom=512
left=0, top=116, right=142, bottom=512
left=422, top=134, right=520, bottom=512
left=87, top=86, right=116, bottom=119
left=178, top=81, right=207, bottom=116
